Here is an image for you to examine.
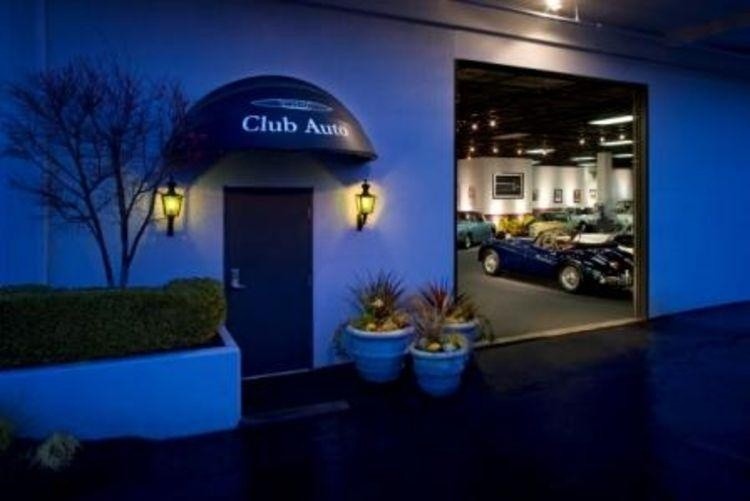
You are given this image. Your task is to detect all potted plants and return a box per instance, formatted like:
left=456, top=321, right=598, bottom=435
left=408, top=307, right=472, bottom=398
left=410, top=279, right=478, bottom=368
left=339, top=268, right=415, bottom=384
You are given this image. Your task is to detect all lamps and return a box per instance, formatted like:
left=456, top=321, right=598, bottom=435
left=162, top=181, right=183, bottom=237
left=355, top=179, right=375, bottom=231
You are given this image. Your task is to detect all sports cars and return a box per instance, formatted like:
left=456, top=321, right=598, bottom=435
left=478, top=230, right=635, bottom=293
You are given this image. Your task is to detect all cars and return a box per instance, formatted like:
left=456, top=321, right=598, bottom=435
left=458, top=210, right=497, bottom=251
left=527, top=197, right=634, bottom=238
